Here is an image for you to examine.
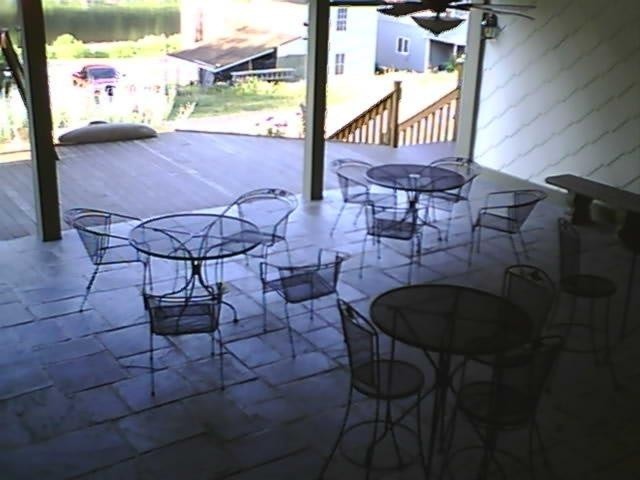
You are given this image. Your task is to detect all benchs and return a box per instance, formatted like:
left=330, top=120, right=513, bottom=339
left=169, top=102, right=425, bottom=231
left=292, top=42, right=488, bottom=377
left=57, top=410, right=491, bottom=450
left=543, top=172, right=640, bottom=239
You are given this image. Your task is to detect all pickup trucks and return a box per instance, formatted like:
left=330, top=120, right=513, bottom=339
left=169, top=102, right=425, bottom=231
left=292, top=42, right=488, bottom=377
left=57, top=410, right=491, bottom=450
left=73, top=65, right=123, bottom=92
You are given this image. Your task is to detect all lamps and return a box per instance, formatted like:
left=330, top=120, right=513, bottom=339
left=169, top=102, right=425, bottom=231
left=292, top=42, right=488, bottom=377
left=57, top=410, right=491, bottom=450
left=480, top=14, right=501, bottom=38
left=410, top=12, right=465, bottom=37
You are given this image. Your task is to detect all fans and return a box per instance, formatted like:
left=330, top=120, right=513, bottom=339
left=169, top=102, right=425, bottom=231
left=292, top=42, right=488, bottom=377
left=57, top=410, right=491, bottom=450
left=324, top=0, right=536, bottom=20
